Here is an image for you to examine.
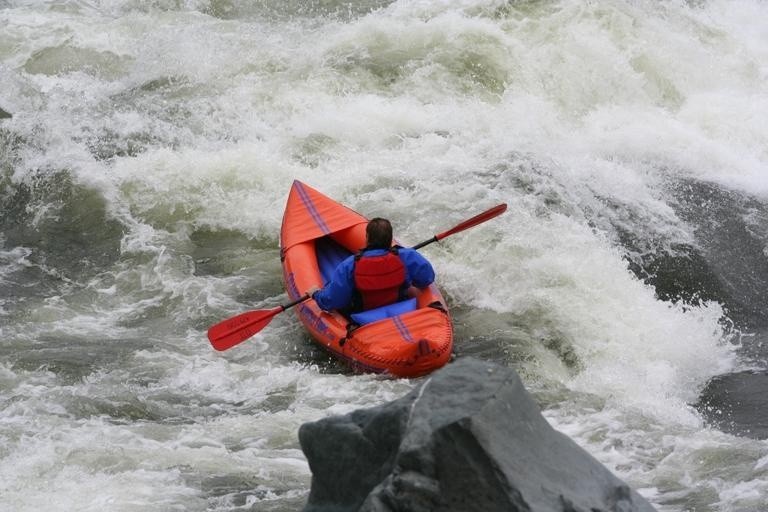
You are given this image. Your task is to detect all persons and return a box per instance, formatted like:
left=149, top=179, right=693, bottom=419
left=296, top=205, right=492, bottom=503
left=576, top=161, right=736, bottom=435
left=305, top=217, right=436, bottom=313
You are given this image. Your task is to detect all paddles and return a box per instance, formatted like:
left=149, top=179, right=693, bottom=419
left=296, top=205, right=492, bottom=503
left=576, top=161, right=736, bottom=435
left=206, top=200, right=507, bottom=351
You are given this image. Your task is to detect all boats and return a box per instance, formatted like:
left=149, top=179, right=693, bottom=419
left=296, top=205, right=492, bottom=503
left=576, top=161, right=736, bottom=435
left=282, top=180, right=455, bottom=376
